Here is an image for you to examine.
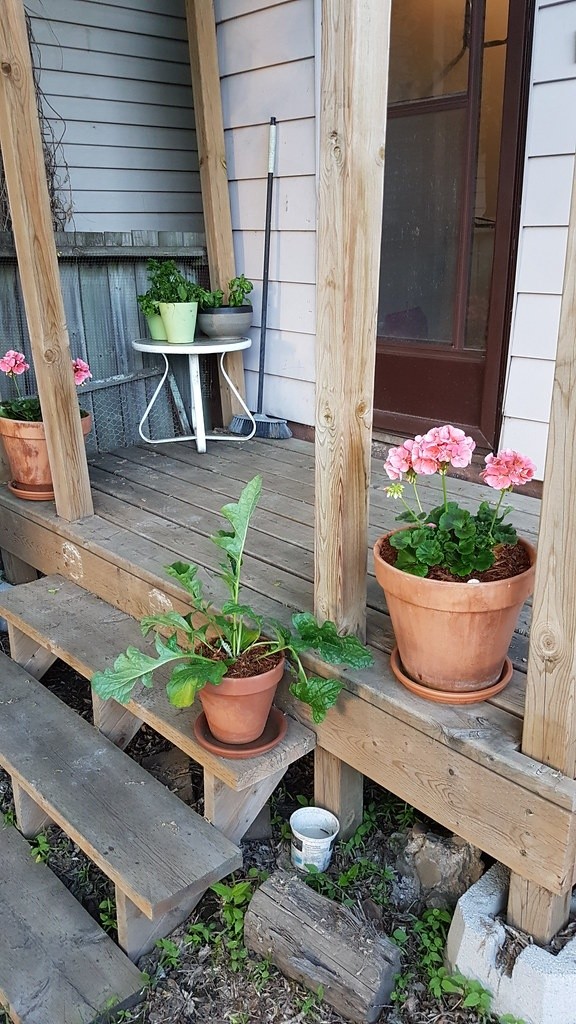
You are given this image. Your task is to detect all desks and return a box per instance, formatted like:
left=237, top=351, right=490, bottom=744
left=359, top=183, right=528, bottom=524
left=131, top=336, right=257, bottom=454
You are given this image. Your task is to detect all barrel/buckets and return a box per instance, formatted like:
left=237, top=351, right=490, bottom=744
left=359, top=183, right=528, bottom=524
left=290, top=806, right=341, bottom=873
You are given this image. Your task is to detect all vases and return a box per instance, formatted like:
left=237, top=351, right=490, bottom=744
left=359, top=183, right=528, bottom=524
left=373, top=525, right=537, bottom=690
left=0, top=399, right=91, bottom=493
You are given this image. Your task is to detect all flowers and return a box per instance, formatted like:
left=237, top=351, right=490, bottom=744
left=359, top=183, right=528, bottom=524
left=0, top=350, right=92, bottom=421
left=381, top=424, right=536, bottom=579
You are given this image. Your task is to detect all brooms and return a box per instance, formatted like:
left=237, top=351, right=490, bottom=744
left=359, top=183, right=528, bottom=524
left=229, top=117, right=293, bottom=439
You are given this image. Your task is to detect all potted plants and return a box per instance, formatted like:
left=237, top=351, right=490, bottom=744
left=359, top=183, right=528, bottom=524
left=90, top=475, right=374, bottom=744
left=197, top=273, right=253, bottom=341
left=145, top=257, right=223, bottom=344
left=136, top=272, right=169, bottom=341
